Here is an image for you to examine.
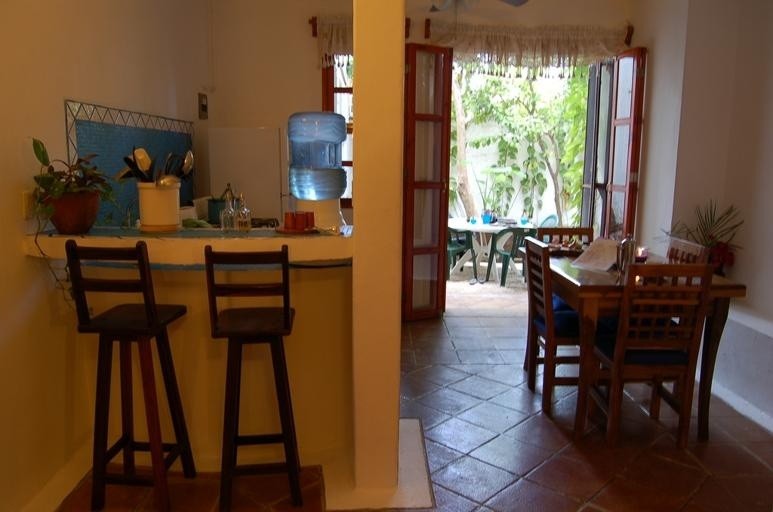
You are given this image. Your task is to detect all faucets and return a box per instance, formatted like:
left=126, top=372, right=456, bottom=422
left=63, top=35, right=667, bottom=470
left=126, top=196, right=138, bottom=227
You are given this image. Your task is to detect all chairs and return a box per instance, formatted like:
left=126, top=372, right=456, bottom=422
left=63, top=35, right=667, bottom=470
left=486, top=228, right=537, bottom=288
left=666, top=237, right=705, bottom=397
left=523, top=236, right=600, bottom=413
left=538, top=228, right=593, bottom=246
left=205, top=244, right=302, bottom=512
left=539, top=215, right=559, bottom=243
left=445, top=212, right=478, bottom=280
left=65, top=239, right=197, bottom=512
left=592, top=263, right=714, bottom=449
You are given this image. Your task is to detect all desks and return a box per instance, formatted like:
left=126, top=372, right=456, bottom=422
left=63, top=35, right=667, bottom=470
left=448, top=217, right=534, bottom=286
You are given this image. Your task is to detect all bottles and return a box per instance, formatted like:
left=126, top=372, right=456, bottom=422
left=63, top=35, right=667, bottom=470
left=520, top=207, right=529, bottom=225
left=217, top=181, right=248, bottom=237
left=465, top=209, right=497, bottom=225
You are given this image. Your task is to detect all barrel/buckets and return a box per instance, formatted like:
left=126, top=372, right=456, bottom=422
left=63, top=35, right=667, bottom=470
left=286, top=111, right=347, bottom=201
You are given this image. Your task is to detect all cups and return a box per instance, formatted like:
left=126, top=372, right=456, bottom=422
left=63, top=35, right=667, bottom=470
left=633, top=246, right=647, bottom=263
left=337, top=225, right=352, bottom=236
left=282, top=211, right=315, bottom=230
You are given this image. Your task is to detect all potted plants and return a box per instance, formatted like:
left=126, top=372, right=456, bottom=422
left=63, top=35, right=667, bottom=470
left=680, top=198, right=745, bottom=278
left=31, top=138, right=116, bottom=234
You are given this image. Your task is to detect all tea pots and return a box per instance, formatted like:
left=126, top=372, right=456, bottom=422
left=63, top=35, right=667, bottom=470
left=614, top=231, right=636, bottom=274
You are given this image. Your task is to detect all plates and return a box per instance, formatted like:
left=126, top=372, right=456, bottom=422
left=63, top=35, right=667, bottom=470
left=274, top=225, right=318, bottom=235
left=498, top=219, right=516, bottom=225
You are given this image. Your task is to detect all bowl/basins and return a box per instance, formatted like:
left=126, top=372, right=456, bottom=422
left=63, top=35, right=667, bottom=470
left=206, top=198, right=240, bottom=223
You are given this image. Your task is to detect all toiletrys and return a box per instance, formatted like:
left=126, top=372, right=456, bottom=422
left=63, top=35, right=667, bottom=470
left=219, top=193, right=235, bottom=230
left=234, top=192, right=251, bottom=232
